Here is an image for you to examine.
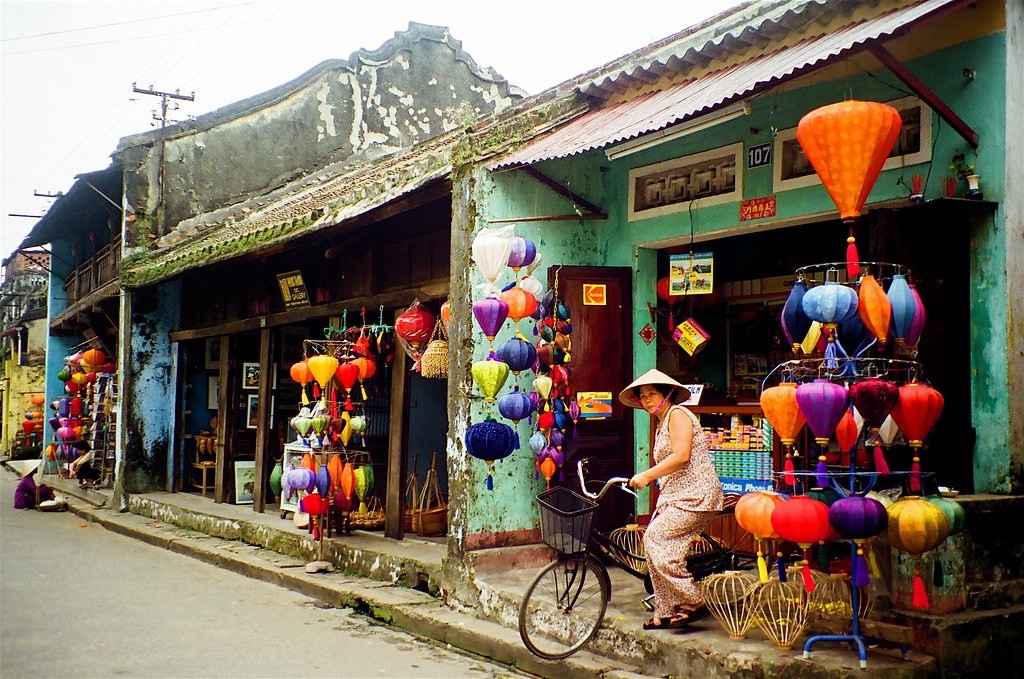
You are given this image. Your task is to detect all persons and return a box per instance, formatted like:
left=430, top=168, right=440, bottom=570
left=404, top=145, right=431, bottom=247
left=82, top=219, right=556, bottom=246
left=14, top=460, right=56, bottom=509
left=619, top=368, right=725, bottom=630
left=69, top=440, right=106, bottom=489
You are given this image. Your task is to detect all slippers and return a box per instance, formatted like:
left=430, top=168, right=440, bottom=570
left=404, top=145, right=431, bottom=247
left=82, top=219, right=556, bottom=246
left=670, top=600, right=711, bottom=629
left=642, top=617, right=670, bottom=629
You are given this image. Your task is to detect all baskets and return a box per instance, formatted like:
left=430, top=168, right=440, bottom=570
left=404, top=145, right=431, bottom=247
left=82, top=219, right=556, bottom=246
left=405, top=505, right=447, bottom=536
left=35, top=502, right=64, bottom=512
left=536, top=486, right=599, bottom=557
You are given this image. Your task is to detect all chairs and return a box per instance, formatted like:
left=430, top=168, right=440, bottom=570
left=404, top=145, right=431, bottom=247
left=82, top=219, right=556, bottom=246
left=188, top=435, right=215, bottom=495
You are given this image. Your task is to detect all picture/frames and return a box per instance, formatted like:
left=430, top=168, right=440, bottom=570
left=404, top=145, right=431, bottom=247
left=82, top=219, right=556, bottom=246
left=246, top=394, right=275, bottom=430
left=235, top=460, right=256, bottom=505
left=205, top=338, right=221, bottom=369
left=287, top=417, right=297, bottom=444
left=208, top=377, right=220, bottom=410
left=241, top=362, right=278, bottom=390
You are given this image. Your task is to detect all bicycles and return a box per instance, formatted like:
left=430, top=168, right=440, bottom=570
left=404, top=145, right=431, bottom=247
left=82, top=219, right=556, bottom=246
left=516, top=462, right=778, bottom=660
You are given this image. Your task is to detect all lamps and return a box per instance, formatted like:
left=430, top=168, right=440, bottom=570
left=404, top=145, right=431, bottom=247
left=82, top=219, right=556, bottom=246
left=604, top=100, right=752, bottom=162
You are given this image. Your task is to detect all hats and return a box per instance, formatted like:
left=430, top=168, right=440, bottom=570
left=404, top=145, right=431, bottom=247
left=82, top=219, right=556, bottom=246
left=618, top=369, right=691, bottom=408
left=21, top=459, right=43, bottom=478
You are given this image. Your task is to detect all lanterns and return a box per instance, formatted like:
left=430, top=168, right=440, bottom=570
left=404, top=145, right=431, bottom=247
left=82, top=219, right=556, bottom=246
left=72, top=243, right=82, bottom=257
left=735, top=270, right=965, bottom=609
left=657, top=275, right=683, bottom=330
left=107, top=214, right=119, bottom=231
left=88, top=226, right=101, bottom=241
left=466, top=229, right=580, bottom=492
left=796, top=100, right=902, bottom=280
left=23, top=349, right=117, bottom=462
left=270, top=301, right=450, bottom=538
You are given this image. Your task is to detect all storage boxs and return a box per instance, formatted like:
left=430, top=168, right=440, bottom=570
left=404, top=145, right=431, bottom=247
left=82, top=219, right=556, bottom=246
left=805, top=273, right=813, bottom=288
left=732, top=281, right=741, bottom=296
left=839, top=269, right=846, bottom=282
left=752, top=279, right=761, bottom=294
left=742, top=280, right=751, bottom=295
left=671, top=317, right=711, bottom=358
left=279, top=326, right=311, bottom=372
left=761, top=275, right=796, bottom=294
left=829, top=271, right=836, bottom=282
left=278, top=371, right=302, bottom=409
left=701, top=414, right=775, bottom=481
left=860, top=266, right=870, bottom=279
left=848, top=274, right=857, bottom=282
left=814, top=272, right=824, bottom=287
left=724, top=282, right=732, bottom=297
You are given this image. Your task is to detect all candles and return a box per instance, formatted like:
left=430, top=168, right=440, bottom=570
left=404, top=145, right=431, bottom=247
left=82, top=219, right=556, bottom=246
left=912, top=173, right=923, bottom=194
left=945, top=176, right=955, bottom=197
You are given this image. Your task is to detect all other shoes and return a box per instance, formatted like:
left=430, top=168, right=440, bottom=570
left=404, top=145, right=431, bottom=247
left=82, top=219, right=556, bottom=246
left=80, top=482, right=96, bottom=489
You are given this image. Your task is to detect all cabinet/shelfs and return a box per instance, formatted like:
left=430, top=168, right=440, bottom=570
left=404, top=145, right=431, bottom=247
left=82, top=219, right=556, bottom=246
left=724, top=288, right=859, bottom=405
left=650, top=405, right=810, bottom=560
left=279, top=443, right=313, bottom=513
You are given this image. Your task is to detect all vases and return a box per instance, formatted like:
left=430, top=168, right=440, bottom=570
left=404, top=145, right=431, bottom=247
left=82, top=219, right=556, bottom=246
left=966, top=174, right=980, bottom=191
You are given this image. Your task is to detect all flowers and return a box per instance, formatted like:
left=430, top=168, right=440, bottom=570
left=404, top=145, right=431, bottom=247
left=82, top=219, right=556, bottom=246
left=950, top=145, right=975, bottom=180
